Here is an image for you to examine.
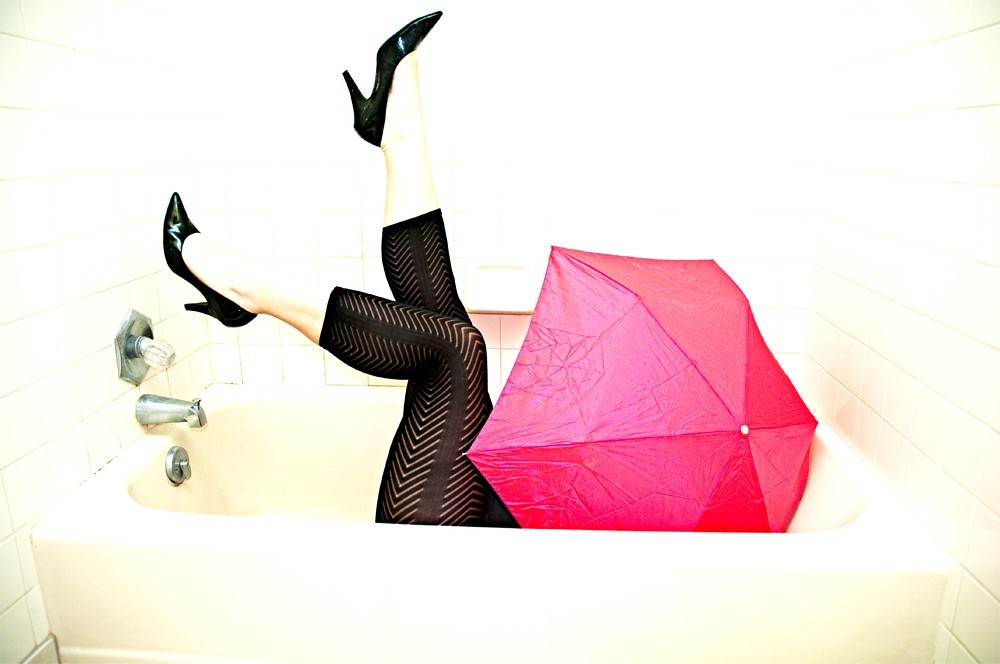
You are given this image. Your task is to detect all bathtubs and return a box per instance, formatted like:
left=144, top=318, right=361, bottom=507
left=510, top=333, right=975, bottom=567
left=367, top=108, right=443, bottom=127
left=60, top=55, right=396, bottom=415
left=26, top=381, right=999, bottom=664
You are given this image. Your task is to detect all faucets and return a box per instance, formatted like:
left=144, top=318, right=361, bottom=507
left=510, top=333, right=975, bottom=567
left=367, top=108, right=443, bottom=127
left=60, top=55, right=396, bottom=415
left=134, top=388, right=209, bottom=430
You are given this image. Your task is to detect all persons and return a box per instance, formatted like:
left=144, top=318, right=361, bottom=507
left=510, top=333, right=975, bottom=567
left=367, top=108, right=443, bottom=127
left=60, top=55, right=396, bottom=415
left=163, top=10, right=521, bottom=530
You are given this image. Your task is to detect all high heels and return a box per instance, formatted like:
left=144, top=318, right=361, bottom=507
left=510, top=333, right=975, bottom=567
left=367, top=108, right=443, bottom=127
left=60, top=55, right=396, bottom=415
left=342, top=9, right=443, bottom=149
left=162, top=190, right=258, bottom=328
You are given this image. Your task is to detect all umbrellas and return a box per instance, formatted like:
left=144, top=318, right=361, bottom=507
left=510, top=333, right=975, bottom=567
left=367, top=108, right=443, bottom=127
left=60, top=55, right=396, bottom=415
left=466, top=247, right=818, bottom=533
